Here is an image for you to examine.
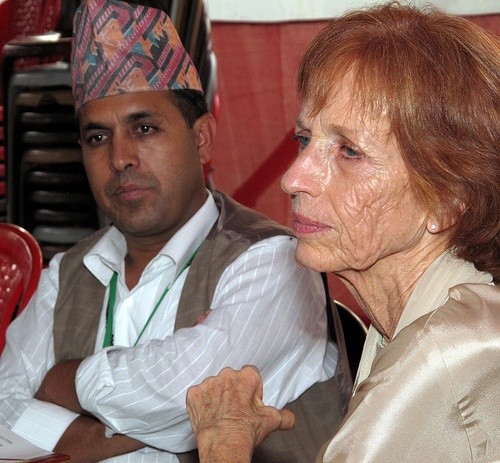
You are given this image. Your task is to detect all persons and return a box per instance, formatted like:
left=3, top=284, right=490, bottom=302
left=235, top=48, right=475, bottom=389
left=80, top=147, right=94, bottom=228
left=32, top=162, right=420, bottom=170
left=186, top=0, right=500, bottom=463
left=0, top=0, right=350, bottom=463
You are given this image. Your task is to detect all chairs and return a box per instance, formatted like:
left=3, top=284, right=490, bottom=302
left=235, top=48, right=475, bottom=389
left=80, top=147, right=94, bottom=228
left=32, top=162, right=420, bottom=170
left=0, top=0, right=211, bottom=364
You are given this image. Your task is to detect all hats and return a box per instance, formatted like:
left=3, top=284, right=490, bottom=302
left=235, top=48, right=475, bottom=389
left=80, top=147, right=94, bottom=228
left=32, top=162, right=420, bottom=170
left=71, top=0, right=204, bottom=113
